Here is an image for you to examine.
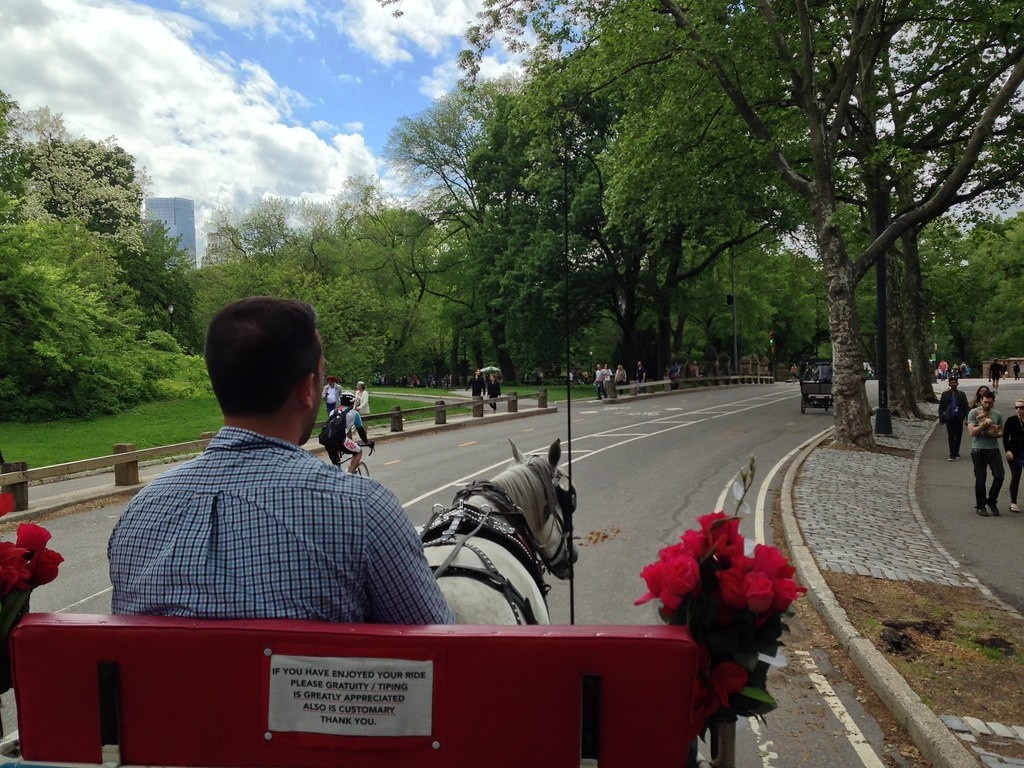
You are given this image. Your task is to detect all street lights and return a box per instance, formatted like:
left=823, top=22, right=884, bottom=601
left=773, top=98, right=897, bottom=439
left=731, top=237, right=783, bottom=385
left=588, top=352, right=593, bottom=383
left=168, top=304, right=174, bottom=335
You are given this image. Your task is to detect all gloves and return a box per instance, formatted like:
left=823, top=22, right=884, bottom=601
left=366, top=440, right=375, bottom=448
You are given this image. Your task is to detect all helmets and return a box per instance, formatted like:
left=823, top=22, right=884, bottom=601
left=340, top=391, right=355, bottom=403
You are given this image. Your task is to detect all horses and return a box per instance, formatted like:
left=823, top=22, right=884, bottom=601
left=418, top=438, right=578, bottom=625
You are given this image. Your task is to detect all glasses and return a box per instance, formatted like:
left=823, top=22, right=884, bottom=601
left=1015, top=405, right=1024, bottom=410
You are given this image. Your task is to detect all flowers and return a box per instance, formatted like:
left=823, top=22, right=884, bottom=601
left=0, top=491, right=66, bottom=739
left=631, top=510, right=809, bottom=744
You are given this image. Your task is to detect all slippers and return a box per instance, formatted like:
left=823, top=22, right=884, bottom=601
left=1009, top=505, right=1020, bottom=513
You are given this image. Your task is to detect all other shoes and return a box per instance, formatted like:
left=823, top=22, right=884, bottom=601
left=987, top=497, right=999, bottom=516
left=950, top=453, right=960, bottom=458
left=976, top=508, right=989, bottom=517
left=948, top=456, right=957, bottom=461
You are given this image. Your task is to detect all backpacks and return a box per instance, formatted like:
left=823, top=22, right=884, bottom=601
left=318, top=405, right=352, bottom=448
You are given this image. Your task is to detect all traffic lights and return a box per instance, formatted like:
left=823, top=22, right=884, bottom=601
left=769, top=332, right=775, bottom=346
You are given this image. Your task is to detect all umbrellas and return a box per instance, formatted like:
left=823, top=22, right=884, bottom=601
left=480, top=366, right=502, bottom=373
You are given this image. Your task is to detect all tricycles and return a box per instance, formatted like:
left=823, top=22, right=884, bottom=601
left=797, top=358, right=834, bottom=414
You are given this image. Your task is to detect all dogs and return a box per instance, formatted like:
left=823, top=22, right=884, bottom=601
left=976, top=411, right=1000, bottom=437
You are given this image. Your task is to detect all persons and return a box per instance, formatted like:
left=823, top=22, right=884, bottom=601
left=989, top=358, right=1003, bottom=393
left=1002, top=398, right=1024, bottom=512
left=790, top=363, right=798, bottom=377
left=329, top=392, right=374, bottom=475
left=593, top=363, right=613, bottom=400
left=351, top=380, right=370, bottom=435
left=966, top=386, right=991, bottom=424
left=968, top=391, right=1006, bottom=517
left=689, top=361, right=700, bottom=387
left=669, top=361, right=680, bottom=389
left=615, top=365, right=627, bottom=396
left=935, top=360, right=971, bottom=381
left=938, top=377, right=968, bottom=460
left=815, top=364, right=832, bottom=381
left=1001, top=360, right=1008, bottom=380
left=322, top=376, right=342, bottom=414
left=1013, top=361, right=1020, bottom=380
left=466, top=369, right=485, bottom=396
left=636, top=361, right=646, bottom=394
left=487, top=374, right=501, bottom=412
left=534, top=369, right=544, bottom=384
left=569, top=371, right=588, bottom=386
left=403, top=373, right=455, bottom=387
left=107, top=295, right=457, bottom=627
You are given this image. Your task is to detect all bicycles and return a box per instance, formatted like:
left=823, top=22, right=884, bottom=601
left=333, top=440, right=376, bottom=478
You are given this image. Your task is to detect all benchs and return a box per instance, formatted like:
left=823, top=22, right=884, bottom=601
left=11, top=611, right=700, bottom=768
left=802, top=381, right=833, bottom=394
